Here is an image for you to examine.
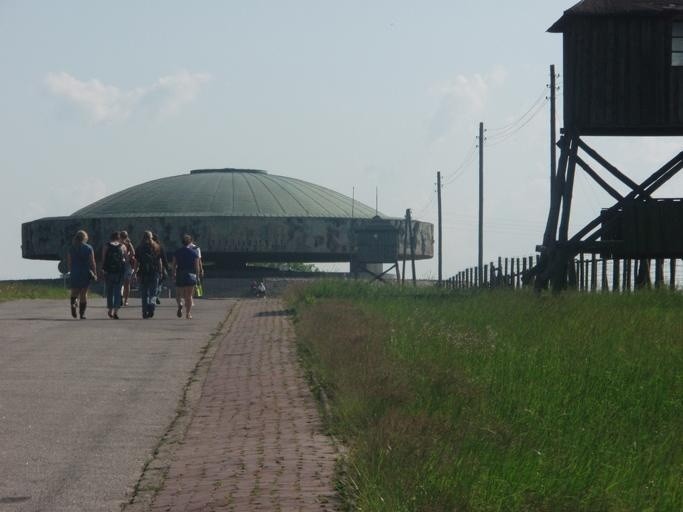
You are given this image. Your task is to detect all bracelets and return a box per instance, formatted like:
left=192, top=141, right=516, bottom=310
left=159, top=272, right=163, bottom=274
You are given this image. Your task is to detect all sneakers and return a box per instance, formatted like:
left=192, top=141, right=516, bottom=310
left=156, top=298, right=160, bottom=304
left=186, top=314, right=192, bottom=319
left=177, top=304, right=182, bottom=318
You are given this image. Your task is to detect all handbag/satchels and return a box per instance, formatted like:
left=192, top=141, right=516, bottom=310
left=192, top=279, right=202, bottom=297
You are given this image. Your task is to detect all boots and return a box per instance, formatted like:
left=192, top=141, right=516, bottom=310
left=79, top=303, right=87, bottom=319
left=71, top=296, right=78, bottom=318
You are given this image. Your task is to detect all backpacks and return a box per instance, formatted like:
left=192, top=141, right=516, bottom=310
left=106, top=242, right=124, bottom=268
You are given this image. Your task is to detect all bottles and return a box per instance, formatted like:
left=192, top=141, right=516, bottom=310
left=196, top=283, right=203, bottom=297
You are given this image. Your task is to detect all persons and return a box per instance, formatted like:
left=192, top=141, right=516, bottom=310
left=132, top=230, right=163, bottom=319
left=152, top=232, right=168, bottom=305
left=102, top=231, right=128, bottom=319
left=67, top=230, right=96, bottom=319
left=120, top=230, right=136, bottom=307
left=171, top=234, right=200, bottom=319
left=250, top=278, right=266, bottom=299
left=190, top=243, right=204, bottom=306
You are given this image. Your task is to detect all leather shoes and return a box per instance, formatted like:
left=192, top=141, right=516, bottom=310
left=143, top=312, right=147, bottom=319
left=148, top=311, right=153, bottom=317
